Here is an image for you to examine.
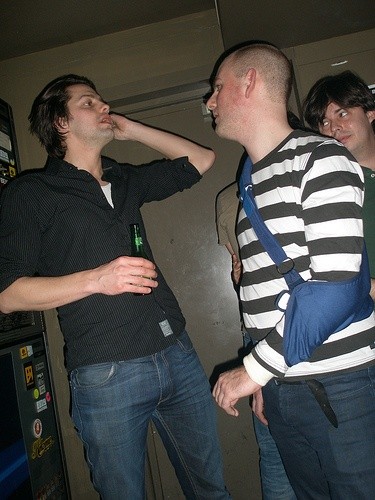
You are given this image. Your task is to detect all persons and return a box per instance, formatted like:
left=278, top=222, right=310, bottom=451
left=298, top=70, right=375, bottom=300
left=205, top=42, right=375, bottom=500
left=215, top=112, right=321, bottom=500
left=0, top=73, right=235, bottom=500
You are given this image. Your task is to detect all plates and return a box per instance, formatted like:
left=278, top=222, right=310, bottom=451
left=129, top=222, right=155, bottom=298
